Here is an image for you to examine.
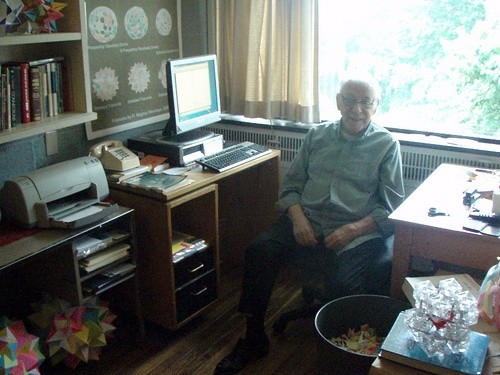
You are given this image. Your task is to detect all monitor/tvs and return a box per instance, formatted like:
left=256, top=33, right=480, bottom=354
left=156, top=54, right=221, bottom=146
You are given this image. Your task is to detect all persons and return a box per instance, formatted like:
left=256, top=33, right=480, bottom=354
left=209, top=71, right=405, bottom=375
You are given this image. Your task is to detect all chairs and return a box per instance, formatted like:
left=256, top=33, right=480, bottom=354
left=273, top=235, right=393, bottom=334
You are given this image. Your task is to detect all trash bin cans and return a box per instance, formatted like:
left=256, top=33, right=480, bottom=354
left=316, top=294, right=412, bottom=375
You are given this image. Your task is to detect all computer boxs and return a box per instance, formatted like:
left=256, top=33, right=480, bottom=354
left=127, top=130, right=225, bottom=166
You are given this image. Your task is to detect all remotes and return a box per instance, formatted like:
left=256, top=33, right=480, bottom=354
left=470, top=212, right=500, bottom=222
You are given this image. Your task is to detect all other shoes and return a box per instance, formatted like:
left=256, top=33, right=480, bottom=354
left=215, top=335, right=269, bottom=375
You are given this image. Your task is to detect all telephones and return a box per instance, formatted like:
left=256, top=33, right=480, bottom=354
left=89, top=139, right=140, bottom=171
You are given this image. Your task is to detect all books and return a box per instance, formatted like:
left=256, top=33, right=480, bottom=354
left=0, top=55, right=72, bottom=134
left=402, top=273, right=499, bottom=334
left=378, top=311, right=490, bottom=375
left=173, top=230, right=208, bottom=264
left=104, top=166, right=191, bottom=194
left=75, top=229, right=136, bottom=297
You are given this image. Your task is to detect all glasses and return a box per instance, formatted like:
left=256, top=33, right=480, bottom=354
left=339, top=94, right=377, bottom=109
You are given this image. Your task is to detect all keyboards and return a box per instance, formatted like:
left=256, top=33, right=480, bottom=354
left=196, top=141, right=274, bottom=173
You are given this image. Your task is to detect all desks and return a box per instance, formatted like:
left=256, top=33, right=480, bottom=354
left=385, top=162, right=500, bottom=299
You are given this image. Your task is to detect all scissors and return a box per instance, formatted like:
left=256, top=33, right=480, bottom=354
left=428, top=206, right=456, bottom=216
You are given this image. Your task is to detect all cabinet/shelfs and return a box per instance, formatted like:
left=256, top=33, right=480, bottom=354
left=0, top=203, right=146, bottom=352
left=0, top=0, right=98, bottom=145
left=111, top=140, right=283, bottom=329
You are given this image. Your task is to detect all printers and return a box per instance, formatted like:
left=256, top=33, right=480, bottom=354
left=0, top=156, right=121, bottom=231
